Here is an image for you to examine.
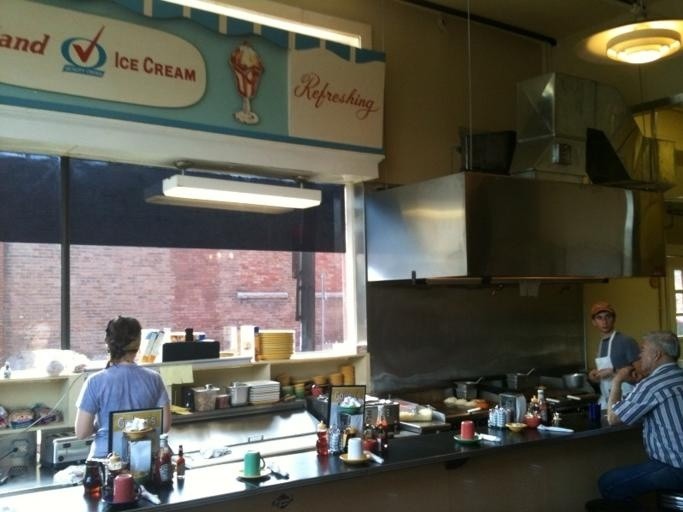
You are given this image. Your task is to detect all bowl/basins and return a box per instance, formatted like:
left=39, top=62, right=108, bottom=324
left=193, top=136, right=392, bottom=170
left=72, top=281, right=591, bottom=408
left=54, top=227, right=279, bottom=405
left=330, top=372, right=342, bottom=386
left=281, top=385, right=294, bottom=396
left=525, top=414, right=541, bottom=428
left=314, top=376, right=327, bottom=384
left=342, top=364, right=353, bottom=386
left=338, top=406, right=362, bottom=414
left=294, top=383, right=305, bottom=398
left=121, top=428, right=153, bottom=440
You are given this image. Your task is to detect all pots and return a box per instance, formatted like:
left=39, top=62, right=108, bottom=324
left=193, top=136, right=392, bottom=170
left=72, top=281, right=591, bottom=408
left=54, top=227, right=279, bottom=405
left=562, top=373, right=585, bottom=391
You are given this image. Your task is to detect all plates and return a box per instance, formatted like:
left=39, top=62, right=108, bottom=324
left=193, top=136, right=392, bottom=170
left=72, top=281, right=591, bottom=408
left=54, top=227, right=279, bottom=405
left=247, top=379, right=280, bottom=405
left=505, top=423, right=528, bottom=433
left=453, top=435, right=482, bottom=447
left=257, top=330, right=295, bottom=361
left=339, top=454, right=370, bottom=465
left=238, top=469, right=272, bottom=479
left=102, top=496, right=140, bottom=507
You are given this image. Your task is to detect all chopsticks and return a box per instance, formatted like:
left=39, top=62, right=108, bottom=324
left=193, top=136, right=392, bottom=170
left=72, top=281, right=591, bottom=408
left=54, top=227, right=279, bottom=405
left=546, top=396, right=561, bottom=404
left=567, top=394, right=580, bottom=401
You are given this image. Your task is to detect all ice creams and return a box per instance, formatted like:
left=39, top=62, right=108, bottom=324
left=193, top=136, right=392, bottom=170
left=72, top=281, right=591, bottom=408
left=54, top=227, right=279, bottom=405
left=228, top=45, right=264, bottom=125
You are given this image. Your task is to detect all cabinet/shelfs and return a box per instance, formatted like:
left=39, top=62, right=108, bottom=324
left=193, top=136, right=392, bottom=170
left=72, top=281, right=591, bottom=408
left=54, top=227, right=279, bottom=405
left=0, top=371, right=97, bottom=468
left=170, top=349, right=364, bottom=419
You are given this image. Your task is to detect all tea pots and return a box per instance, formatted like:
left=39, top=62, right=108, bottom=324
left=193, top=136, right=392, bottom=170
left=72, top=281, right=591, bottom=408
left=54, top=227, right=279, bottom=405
left=82, top=459, right=105, bottom=490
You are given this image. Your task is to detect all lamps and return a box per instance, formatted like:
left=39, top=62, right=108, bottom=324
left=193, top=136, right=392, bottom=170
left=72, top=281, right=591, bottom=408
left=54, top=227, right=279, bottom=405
left=574, top=2, right=683, bottom=68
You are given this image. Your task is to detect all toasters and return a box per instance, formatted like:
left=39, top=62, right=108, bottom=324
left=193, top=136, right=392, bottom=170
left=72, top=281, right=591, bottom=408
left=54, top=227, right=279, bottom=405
left=43, top=431, right=100, bottom=466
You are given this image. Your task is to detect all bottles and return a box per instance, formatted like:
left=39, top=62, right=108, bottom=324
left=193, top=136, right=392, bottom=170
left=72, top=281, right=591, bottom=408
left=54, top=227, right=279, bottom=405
left=82, top=461, right=102, bottom=497
left=528, top=395, right=539, bottom=416
left=107, top=452, right=123, bottom=496
left=344, top=429, right=357, bottom=453
left=376, top=424, right=386, bottom=453
left=155, top=434, right=175, bottom=488
left=177, top=444, right=185, bottom=478
left=537, top=388, right=548, bottom=423
left=316, top=420, right=328, bottom=456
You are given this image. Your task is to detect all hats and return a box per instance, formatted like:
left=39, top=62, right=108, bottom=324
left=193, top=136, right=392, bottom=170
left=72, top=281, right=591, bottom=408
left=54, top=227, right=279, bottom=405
left=591, top=300, right=616, bottom=317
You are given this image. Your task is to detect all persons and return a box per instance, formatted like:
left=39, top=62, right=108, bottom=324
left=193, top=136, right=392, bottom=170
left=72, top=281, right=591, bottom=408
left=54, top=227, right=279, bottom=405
left=597, top=330, right=683, bottom=512
left=75, top=314, right=172, bottom=462
left=589, top=301, right=641, bottom=411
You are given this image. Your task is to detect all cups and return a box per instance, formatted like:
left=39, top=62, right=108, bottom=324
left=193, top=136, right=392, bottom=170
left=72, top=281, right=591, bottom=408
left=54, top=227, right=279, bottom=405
left=112, top=474, right=142, bottom=502
left=461, top=421, right=474, bottom=439
left=244, top=450, right=265, bottom=476
left=217, top=395, right=231, bottom=409
left=347, top=438, right=362, bottom=459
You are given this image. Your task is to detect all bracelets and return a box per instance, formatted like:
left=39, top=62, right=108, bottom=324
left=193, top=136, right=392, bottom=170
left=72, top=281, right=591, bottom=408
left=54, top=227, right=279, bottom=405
left=612, top=367, right=616, bottom=375
